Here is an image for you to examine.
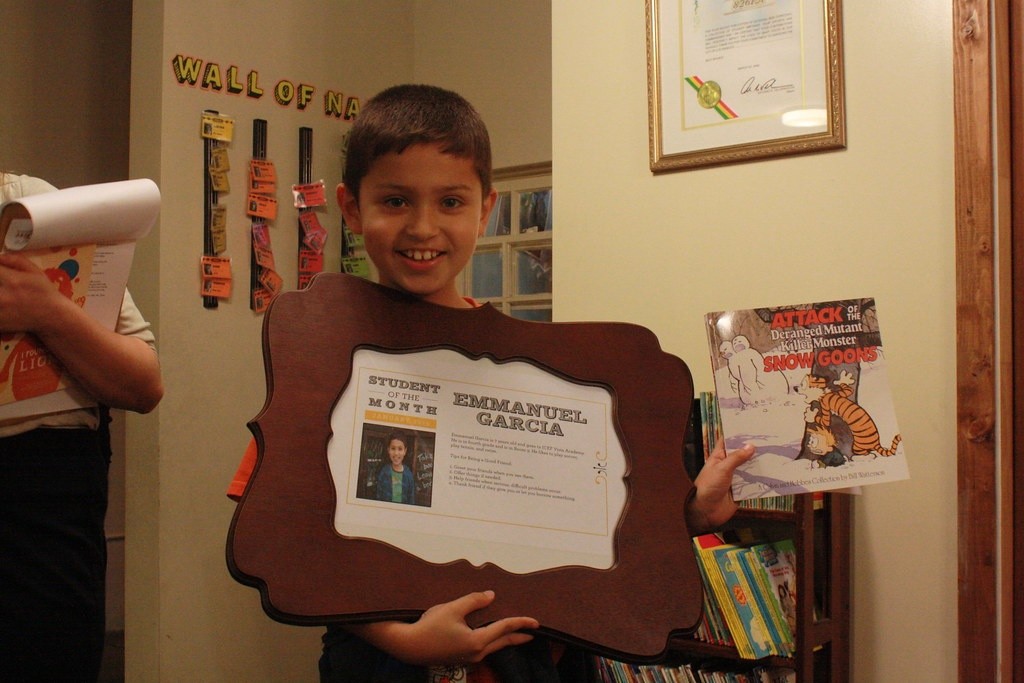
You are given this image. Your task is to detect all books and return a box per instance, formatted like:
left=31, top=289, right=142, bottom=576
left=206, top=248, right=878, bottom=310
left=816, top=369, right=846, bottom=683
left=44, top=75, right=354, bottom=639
left=700, top=392, right=823, bottom=513
left=703, top=297, right=912, bottom=502
left=691, top=527, right=824, bottom=661
left=0, top=178, right=161, bottom=440
left=596, top=656, right=799, bottom=683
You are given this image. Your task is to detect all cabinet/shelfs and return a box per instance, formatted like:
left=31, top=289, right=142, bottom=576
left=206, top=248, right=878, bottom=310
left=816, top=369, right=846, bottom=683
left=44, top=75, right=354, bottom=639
left=495, top=392, right=855, bottom=683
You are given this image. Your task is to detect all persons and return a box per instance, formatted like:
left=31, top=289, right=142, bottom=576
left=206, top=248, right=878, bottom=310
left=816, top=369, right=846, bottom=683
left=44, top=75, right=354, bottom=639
left=377, top=433, right=415, bottom=508
left=0, top=172, right=167, bottom=683
left=226, top=82, right=755, bottom=681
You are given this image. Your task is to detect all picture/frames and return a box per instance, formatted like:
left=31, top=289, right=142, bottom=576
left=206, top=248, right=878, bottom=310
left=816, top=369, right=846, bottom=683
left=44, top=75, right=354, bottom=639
left=645, top=0, right=848, bottom=172
left=223, top=270, right=704, bottom=665
